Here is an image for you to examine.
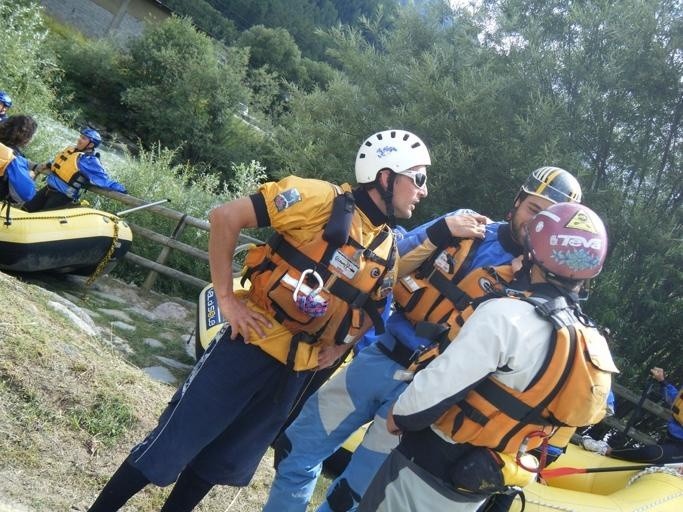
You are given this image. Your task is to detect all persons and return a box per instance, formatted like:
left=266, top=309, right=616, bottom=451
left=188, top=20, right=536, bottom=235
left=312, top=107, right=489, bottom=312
left=0, top=90, right=129, bottom=212
left=88, top=130, right=682, bottom=509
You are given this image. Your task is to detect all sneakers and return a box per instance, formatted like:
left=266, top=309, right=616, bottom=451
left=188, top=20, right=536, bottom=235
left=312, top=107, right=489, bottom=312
left=584, top=439, right=610, bottom=456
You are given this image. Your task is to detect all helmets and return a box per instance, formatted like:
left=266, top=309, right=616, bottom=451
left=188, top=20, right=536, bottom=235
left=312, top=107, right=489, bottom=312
left=513, top=167, right=608, bottom=280
left=0, top=93, right=12, bottom=108
left=80, top=128, right=102, bottom=148
left=354, top=129, right=432, bottom=184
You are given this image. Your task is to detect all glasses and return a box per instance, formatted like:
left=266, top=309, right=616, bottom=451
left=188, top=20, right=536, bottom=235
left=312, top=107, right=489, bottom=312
left=399, top=169, right=427, bottom=189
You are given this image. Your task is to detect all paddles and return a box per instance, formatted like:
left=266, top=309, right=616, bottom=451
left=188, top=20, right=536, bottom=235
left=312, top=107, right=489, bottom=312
left=606, top=373, right=654, bottom=450
left=540, top=463, right=683, bottom=479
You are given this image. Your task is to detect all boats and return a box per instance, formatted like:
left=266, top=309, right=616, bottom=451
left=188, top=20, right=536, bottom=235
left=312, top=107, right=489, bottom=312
left=190, top=274, right=683, bottom=509
left=0, top=197, right=133, bottom=282
